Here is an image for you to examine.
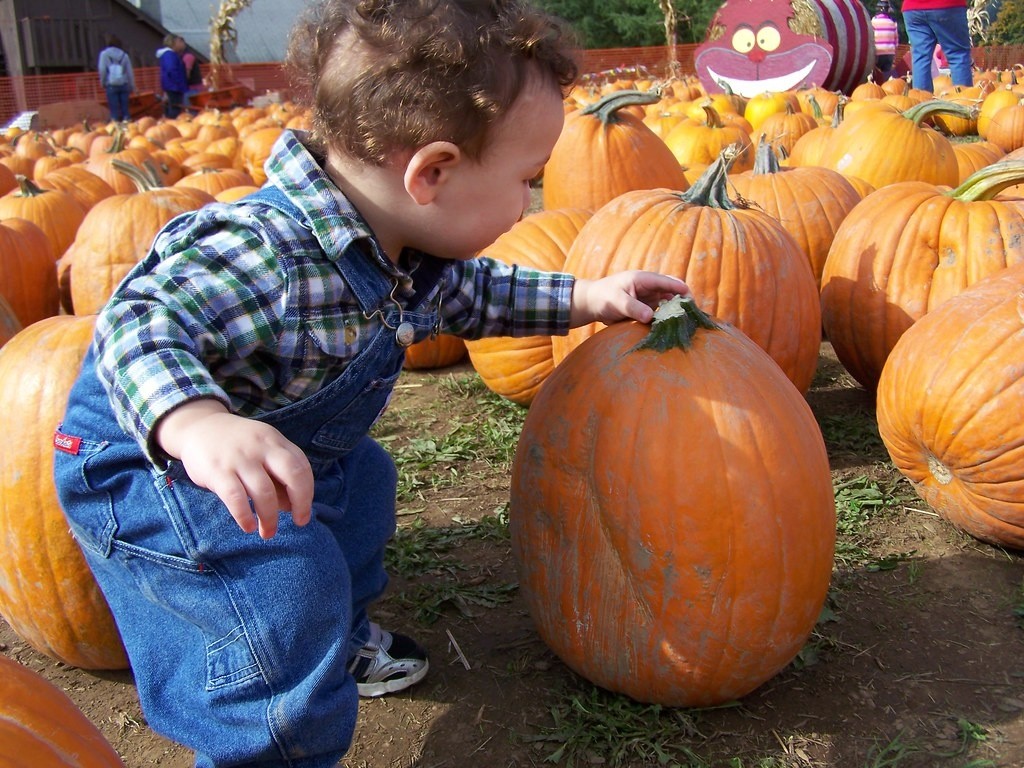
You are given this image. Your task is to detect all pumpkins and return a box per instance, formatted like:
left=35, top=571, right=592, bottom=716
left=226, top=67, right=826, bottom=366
left=0, top=654, right=127, bottom=768
left=0, top=65, right=1023, bottom=706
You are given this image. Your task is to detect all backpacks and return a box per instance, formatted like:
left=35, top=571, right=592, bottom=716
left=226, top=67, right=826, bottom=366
left=103, top=49, right=126, bottom=86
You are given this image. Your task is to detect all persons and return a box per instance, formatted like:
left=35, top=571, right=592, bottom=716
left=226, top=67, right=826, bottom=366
left=156, top=34, right=203, bottom=118
left=55, top=0, right=689, bottom=768
left=871, top=0, right=898, bottom=72
left=98, top=32, right=135, bottom=121
left=901, top=0, right=974, bottom=95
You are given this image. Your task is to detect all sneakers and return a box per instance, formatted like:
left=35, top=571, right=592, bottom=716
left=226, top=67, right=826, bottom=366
left=345, top=622, right=429, bottom=697
